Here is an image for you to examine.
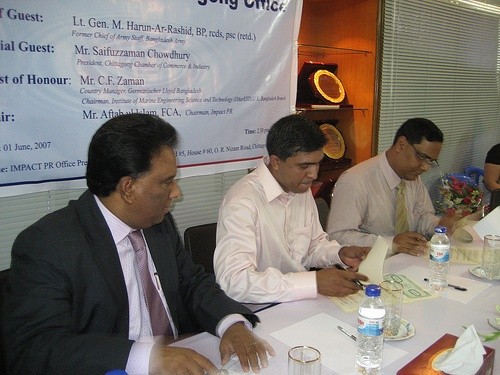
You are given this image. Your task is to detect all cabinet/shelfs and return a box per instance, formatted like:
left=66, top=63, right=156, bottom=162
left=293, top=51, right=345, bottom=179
left=296, top=41, right=373, bottom=166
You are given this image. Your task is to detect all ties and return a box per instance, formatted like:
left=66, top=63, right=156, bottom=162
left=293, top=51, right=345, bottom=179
left=394, top=180, right=409, bottom=237
left=128, top=229, right=178, bottom=345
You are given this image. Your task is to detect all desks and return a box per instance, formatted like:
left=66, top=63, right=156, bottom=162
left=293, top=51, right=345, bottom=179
left=109, top=240, right=500, bottom=375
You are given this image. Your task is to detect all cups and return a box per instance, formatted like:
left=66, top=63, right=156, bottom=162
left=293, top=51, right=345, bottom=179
left=379, top=280, right=403, bottom=337
left=481, top=234, right=500, bottom=276
left=288, top=346, right=322, bottom=375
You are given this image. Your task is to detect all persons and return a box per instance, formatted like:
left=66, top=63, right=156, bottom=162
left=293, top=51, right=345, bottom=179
left=483, top=143, right=500, bottom=212
left=325, top=117, right=461, bottom=261
left=0, top=113, right=275, bottom=375
left=212, top=114, right=373, bottom=305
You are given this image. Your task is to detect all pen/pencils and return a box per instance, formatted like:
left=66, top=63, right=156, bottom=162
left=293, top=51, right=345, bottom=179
left=335, top=263, right=363, bottom=286
left=424, top=278, right=467, bottom=291
left=338, top=326, right=356, bottom=340
left=416, top=238, right=424, bottom=244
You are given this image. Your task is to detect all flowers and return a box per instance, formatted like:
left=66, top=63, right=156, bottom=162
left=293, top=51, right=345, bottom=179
left=429, top=174, right=487, bottom=221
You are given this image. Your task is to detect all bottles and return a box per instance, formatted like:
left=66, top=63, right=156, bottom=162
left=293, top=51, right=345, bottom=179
left=355, top=284, right=386, bottom=375
left=428, top=225, right=450, bottom=291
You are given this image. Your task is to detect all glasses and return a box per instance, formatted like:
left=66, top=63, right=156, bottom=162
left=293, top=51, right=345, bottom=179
left=410, top=142, right=439, bottom=168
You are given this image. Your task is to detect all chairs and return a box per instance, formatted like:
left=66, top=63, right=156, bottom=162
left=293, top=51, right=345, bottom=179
left=0, top=167, right=484, bottom=375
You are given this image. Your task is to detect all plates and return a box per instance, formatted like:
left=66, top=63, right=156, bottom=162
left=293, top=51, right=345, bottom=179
left=383, top=314, right=416, bottom=341
left=469, top=265, right=500, bottom=280
